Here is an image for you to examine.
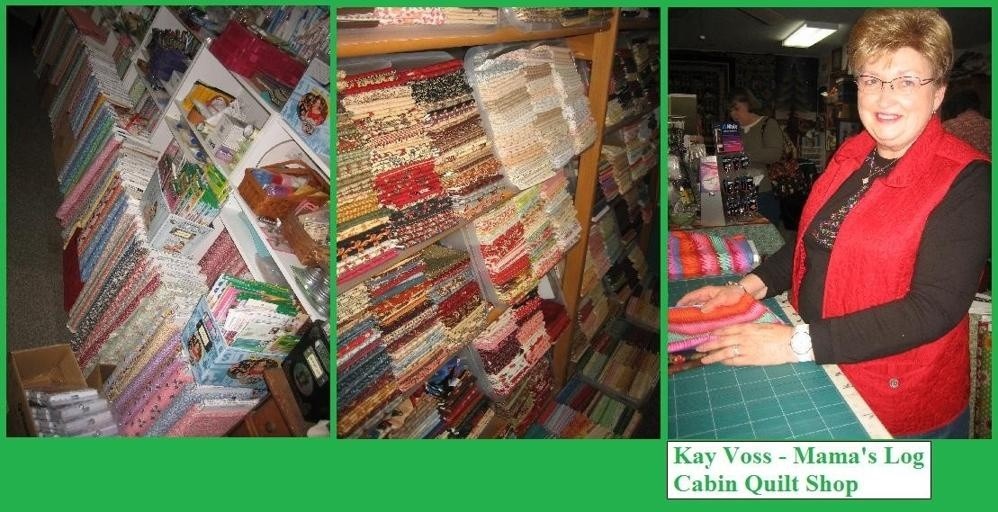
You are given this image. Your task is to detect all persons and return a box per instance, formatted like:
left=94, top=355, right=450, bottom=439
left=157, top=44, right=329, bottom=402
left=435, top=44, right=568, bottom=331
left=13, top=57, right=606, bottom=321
left=711, top=85, right=785, bottom=235
left=674, top=7, right=992, bottom=440
left=939, top=88, right=992, bottom=162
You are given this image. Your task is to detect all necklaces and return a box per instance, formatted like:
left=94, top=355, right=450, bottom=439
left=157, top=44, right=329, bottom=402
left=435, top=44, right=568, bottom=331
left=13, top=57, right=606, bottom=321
left=862, top=144, right=908, bottom=184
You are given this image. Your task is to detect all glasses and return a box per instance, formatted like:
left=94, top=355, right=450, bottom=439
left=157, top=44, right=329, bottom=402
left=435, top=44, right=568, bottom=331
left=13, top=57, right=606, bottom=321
left=852, top=74, right=935, bottom=95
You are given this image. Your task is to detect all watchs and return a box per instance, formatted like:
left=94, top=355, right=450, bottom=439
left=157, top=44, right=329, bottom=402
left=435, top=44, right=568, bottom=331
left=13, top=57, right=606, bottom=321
left=790, top=323, right=815, bottom=364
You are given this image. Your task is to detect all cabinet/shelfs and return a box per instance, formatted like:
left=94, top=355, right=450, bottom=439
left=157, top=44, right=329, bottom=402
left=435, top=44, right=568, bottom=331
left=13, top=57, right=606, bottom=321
left=337, top=6, right=660, bottom=439
left=38, top=5, right=331, bottom=327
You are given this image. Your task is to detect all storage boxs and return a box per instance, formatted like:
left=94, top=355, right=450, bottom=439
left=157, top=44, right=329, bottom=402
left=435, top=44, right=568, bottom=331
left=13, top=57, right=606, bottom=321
left=9, top=346, right=91, bottom=435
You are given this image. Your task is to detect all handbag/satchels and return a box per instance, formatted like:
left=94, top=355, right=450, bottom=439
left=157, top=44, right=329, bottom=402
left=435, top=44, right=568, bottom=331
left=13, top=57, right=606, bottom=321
left=768, top=156, right=810, bottom=202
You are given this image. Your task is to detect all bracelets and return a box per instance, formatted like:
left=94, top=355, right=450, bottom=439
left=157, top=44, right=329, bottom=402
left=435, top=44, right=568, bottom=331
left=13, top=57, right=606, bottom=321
left=725, top=280, right=749, bottom=295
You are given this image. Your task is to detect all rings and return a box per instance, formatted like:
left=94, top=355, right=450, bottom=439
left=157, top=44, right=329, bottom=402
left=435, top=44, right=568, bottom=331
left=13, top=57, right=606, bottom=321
left=733, top=345, right=742, bottom=357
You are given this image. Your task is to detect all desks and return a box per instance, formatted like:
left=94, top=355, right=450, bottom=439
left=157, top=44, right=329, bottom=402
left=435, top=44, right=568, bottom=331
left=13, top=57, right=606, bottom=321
left=665, top=211, right=893, bottom=442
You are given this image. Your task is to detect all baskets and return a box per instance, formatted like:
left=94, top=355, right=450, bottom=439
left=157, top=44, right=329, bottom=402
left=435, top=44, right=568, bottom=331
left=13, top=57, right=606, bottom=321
left=237, top=158, right=333, bottom=269
